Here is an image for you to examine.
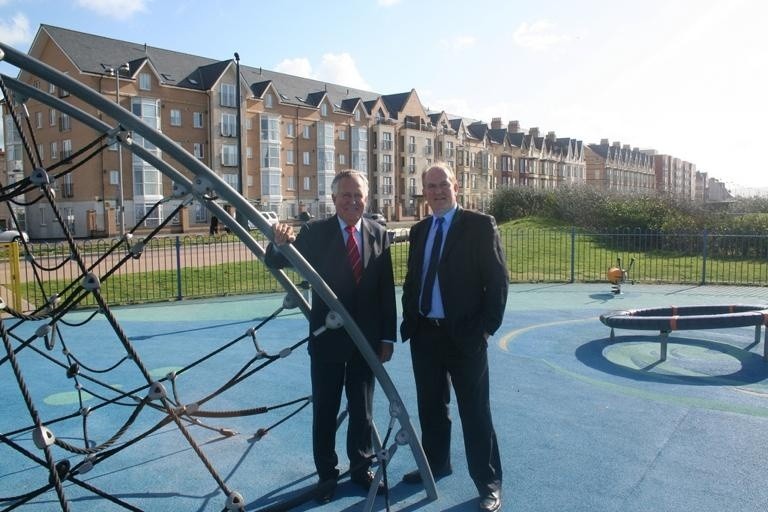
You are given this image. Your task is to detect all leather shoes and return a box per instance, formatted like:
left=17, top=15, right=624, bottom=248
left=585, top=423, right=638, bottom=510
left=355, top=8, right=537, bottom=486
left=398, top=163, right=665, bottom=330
left=479, top=486, right=502, bottom=512
left=350, top=471, right=388, bottom=495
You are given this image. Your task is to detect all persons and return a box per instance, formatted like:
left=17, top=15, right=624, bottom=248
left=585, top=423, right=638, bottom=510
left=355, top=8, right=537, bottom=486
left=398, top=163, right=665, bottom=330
left=271, top=169, right=396, bottom=505
left=400, top=161, right=509, bottom=512
left=210, top=216, right=218, bottom=236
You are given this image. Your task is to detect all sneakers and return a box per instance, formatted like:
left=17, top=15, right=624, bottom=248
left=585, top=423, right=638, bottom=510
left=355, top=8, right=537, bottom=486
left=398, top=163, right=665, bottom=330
left=402, top=465, right=453, bottom=484
left=315, top=478, right=338, bottom=504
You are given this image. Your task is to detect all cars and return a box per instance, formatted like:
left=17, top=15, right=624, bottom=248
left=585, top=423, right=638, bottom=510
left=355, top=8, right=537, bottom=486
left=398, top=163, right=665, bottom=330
left=224, top=211, right=279, bottom=234
left=363, top=214, right=387, bottom=226
left=0, top=230, right=29, bottom=243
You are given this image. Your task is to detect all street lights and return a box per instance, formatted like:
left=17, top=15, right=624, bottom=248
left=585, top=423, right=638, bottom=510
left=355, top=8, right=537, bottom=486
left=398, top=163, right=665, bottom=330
left=107, top=62, right=131, bottom=240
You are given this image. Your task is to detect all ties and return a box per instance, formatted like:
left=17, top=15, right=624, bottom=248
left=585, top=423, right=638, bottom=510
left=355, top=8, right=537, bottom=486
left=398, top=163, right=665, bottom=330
left=343, top=224, right=362, bottom=287
left=420, top=217, right=445, bottom=316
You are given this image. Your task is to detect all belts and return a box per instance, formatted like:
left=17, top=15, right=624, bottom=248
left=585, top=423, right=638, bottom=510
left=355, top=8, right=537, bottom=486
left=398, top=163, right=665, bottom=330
left=425, top=318, right=448, bottom=327
